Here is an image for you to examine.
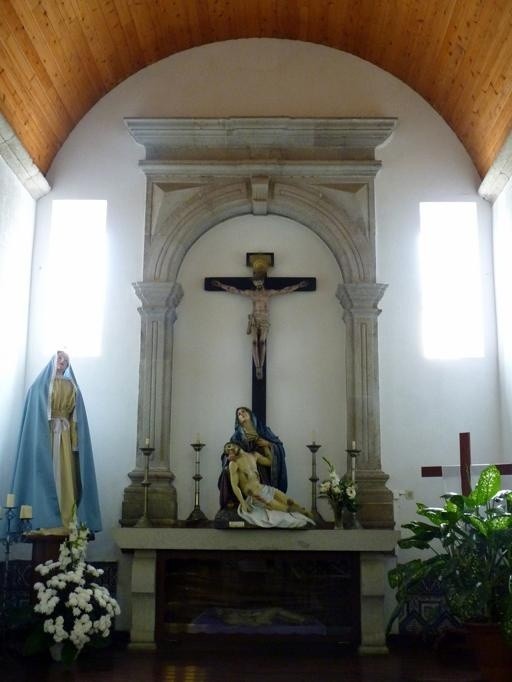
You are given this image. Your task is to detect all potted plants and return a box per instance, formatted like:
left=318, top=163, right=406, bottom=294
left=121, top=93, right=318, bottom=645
left=384, top=463, right=511, bottom=681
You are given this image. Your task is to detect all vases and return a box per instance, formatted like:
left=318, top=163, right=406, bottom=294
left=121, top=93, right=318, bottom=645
left=48, top=637, right=82, bottom=663
left=328, top=500, right=344, bottom=529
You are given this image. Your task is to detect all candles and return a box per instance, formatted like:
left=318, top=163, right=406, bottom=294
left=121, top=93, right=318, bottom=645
left=194, top=431, right=201, bottom=444
left=350, top=440, right=357, bottom=449
left=7, top=492, right=15, bottom=508
left=310, top=429, right=316, bottom=442
left=20, top=504, right=33, bottom=519
left=145, top=437, right=150, bottom=444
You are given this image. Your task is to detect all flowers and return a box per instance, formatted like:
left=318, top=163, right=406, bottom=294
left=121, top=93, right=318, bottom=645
left=317, top=455, right=364, bottom=526
left=10, top=497, right=121, bottom=662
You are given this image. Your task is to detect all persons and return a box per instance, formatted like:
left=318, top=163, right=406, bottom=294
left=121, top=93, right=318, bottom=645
left=223, top=437, right=314, bottom=519
left=216, top=406, right=288, bottom=513
left=7, top=350, right=103, bottom=540
left=209, top=274, right=308, bottom=382
